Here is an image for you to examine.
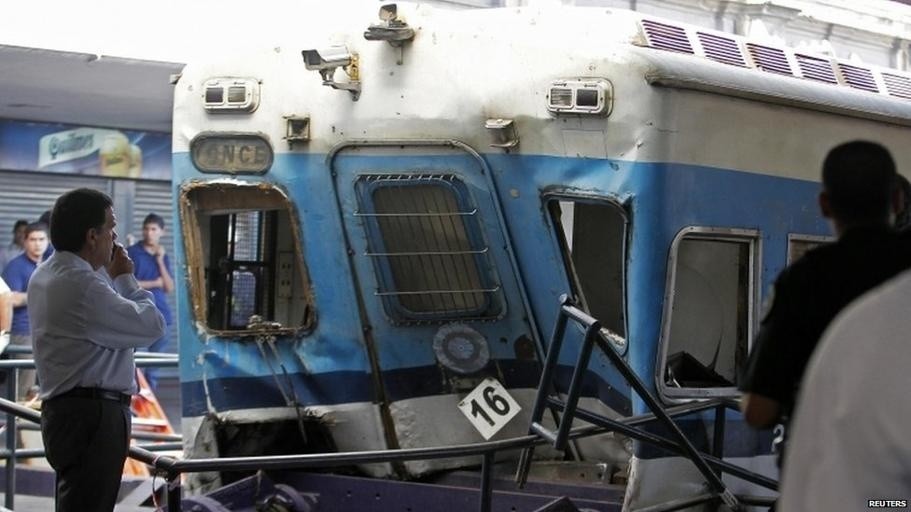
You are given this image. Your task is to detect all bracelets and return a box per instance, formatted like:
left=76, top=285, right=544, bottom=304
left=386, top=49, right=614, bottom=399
left=158, top=277, right=163, bottom=288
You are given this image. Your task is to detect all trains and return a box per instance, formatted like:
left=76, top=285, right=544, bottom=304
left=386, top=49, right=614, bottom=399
left=170, top=0, right=910, bottom=510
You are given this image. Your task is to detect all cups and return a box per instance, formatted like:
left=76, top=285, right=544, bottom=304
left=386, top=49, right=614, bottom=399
left=99, top=133, right=143, bottom=179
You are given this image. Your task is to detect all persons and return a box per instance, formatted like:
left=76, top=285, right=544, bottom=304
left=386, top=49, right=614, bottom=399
left=772, top=263, right=911, bottom=512
left=884, top=172, right=911, bottom=230
left=0, top=208, right=53, bottom=465
left=126, top=211, right=176, bottom=407
left=26, top=185, right=171, bottom=511
left=0, top=275, right=15, bottom=360
left=733, top=138, right=911, bottom=461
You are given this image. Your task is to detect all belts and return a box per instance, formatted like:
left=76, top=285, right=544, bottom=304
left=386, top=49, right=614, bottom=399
left=68, top=386, right=131, bottom=405
